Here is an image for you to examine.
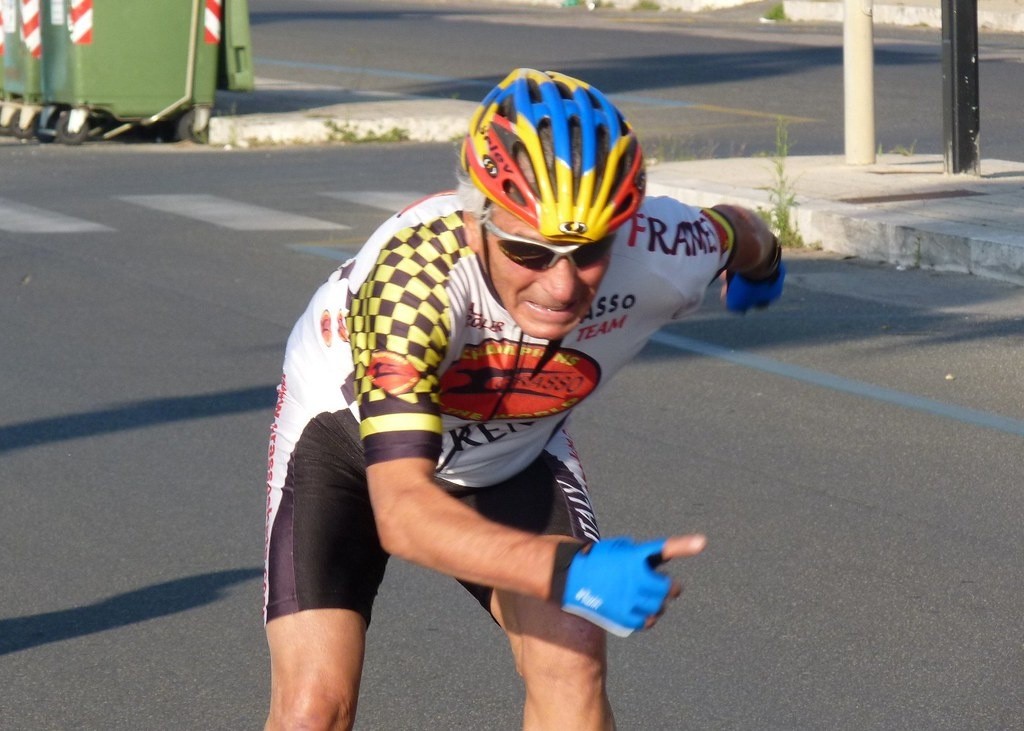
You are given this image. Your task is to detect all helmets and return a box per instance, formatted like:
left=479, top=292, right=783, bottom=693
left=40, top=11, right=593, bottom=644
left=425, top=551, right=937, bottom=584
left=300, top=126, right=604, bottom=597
left=460, top=69, right=646, bottom=244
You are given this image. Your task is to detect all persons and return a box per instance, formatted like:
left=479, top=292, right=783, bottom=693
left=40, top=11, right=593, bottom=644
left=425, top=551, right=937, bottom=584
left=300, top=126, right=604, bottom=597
left=260, top=67, right=781, bottom=731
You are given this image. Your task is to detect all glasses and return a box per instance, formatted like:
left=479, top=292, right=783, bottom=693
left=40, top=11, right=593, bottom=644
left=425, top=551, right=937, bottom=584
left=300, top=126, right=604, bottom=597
left=481, top=212, right=616, bottom=273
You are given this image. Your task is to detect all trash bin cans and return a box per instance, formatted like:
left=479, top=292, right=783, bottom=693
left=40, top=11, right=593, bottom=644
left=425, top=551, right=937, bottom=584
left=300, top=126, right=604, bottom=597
left=0, top=0, right=256, bottom=146
